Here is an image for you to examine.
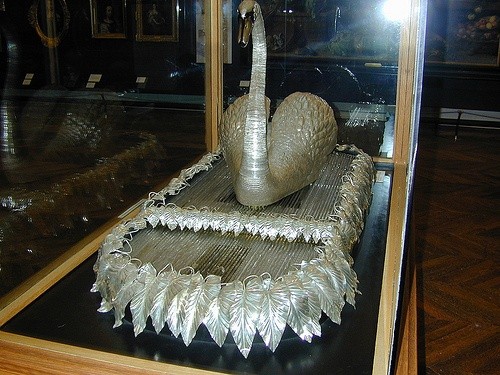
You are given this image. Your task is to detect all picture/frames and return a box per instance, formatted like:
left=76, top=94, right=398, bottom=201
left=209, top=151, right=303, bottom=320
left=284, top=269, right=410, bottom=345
left=90, top=0, right=127, bottom=40
left=135, top=0, right=178, bottom=43
left=267, top=0, right=499, bottom=68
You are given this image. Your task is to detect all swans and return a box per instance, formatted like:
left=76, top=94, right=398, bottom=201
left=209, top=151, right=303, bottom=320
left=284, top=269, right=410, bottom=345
left=216, top=0, right=339, bottom=208
